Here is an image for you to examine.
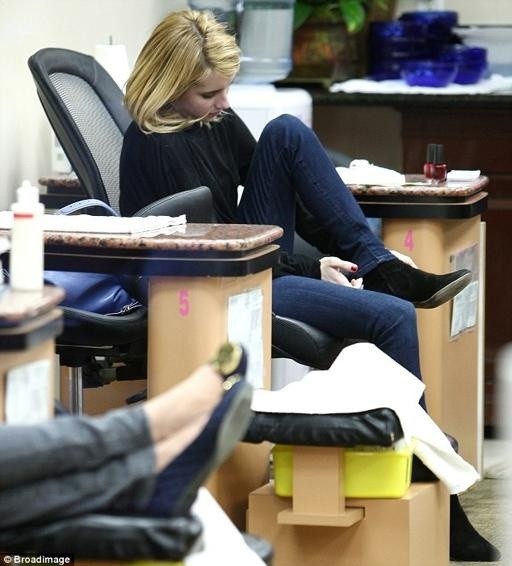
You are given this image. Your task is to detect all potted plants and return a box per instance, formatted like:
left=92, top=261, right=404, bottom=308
left=293, top=0, right=370, bottom=84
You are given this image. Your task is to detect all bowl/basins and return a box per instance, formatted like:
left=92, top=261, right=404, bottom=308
left=360, top=8, right=488, bottom=87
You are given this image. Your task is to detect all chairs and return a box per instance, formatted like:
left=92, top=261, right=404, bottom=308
left=29, top=48, right=343, bottom=369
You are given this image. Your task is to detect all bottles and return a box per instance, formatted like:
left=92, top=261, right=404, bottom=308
left=422, top=143, right=447, bottom=187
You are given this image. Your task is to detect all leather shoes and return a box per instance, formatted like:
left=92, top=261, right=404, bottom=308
left=141, top=340, right=254, bottom=516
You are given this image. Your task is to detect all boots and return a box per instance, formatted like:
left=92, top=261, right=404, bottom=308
left=450, top=494, right=502, bottom=561
left=362, top=258, right=473, bottom=309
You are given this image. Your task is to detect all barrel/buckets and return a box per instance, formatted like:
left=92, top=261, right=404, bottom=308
left=191, top=0, right=294, bottom=86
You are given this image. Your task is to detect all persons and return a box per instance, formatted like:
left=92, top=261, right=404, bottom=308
left=1, top=341, right=255, bottom=550
left=116, top=4, right=504, bottom=565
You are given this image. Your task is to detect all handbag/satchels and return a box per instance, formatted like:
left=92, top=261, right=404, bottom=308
left=44, top=270, right=144, bottom=316
